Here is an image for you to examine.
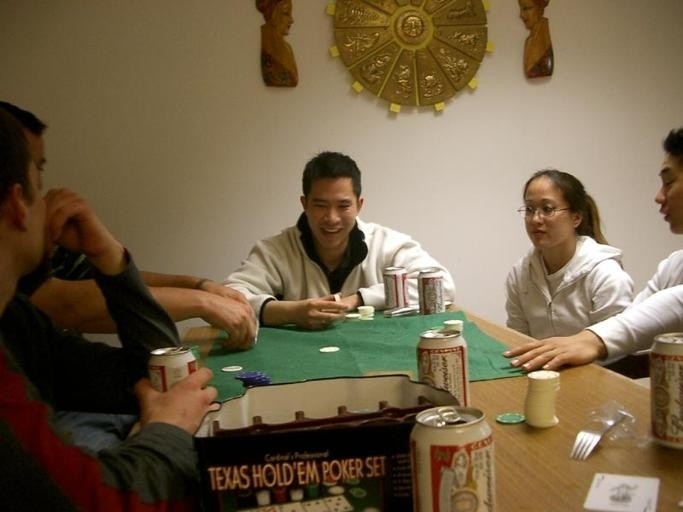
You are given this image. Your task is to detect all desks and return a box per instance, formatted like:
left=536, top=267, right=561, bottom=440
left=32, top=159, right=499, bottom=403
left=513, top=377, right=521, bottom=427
left=127, top=304, right=683, bottom=511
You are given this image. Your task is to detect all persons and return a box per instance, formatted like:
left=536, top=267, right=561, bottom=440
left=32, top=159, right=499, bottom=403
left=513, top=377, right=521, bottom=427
left=223, top=152, right=455, bottom=330
left=519, top=0, right=553, bottom=78
left=0, top=103, right=258, bottom=512
left=503, top=127, right=682, bottom=372
left=256, top=0, right=298, bottom=87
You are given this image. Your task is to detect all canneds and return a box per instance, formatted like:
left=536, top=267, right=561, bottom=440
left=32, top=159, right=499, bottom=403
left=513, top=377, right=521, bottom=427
left=416, top=329, right=469, bottom=407
left=417, top=266, right=446, bottom=315
left=147, top=346, right=197, bottom=393
left=382, top=265, right=409, bottom=310
left=409, top=405, right=496, bottom=512
left=649, top=332, right=683, bottom=450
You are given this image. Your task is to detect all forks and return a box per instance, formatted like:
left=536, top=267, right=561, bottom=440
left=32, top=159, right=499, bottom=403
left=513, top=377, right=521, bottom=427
left=569, top=412, right=627, bottom=460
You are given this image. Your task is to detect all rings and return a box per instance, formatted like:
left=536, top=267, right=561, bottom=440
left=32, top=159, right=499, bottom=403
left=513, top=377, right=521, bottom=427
left=321, top=319, right=326, bottom=327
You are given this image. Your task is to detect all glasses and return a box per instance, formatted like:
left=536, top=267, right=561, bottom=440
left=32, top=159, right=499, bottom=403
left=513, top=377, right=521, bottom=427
left=517, top=204, right=572, bottom=218
